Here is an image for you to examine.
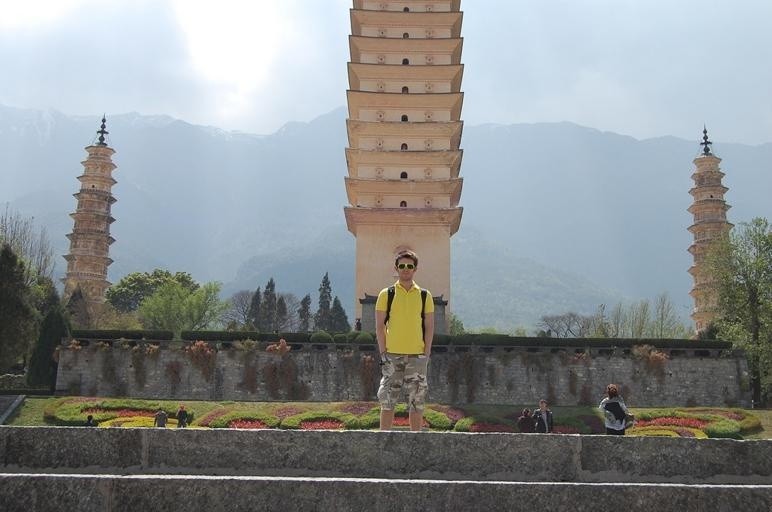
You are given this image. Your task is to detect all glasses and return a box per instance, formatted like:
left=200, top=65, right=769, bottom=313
left=395, top=263, right=415, bottom=272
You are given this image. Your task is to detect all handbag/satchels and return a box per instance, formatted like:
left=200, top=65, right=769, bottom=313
left=626, top=413, right=634, bottom=428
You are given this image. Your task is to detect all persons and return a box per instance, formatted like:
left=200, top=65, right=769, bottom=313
left=532, top=399, right=553, bottom=433
left=153, top=407, right=168, bottom=427
left=176, top=404, right=188, bottom=428
left=373, top=250, right=436, bottom=432
left=517, top=407, right=535, bottom=433
left=597, top=383, right=628, bottom=435
left=84, top=414, right=95, bottom=426
